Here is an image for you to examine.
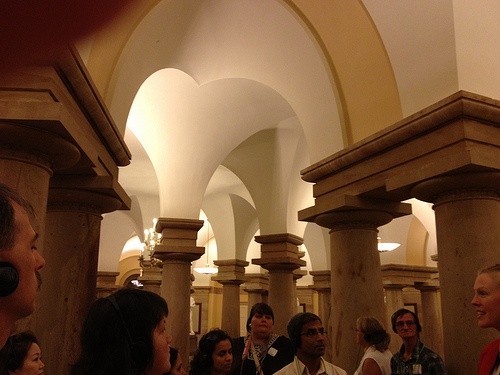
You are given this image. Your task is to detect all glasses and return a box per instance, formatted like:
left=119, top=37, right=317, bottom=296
left=300, top=329, right=328, bottom=337
left=355, top=328, right=364, bottom=332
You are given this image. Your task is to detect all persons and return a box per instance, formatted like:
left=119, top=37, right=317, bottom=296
left=390, top=308, right=447, bottom=375
left=272, top=312, right=348, bottom=375
left=353, top=316, right=393, bottom=375
left=0, top=179, right=47, bottom=351
left=231, top=302, right=296, bottom=375
left=0, top=332, right=45, bottom=375
left=69, top=287, right=172, bottom=375
left=189, top=328, right=234, bottom=375
left=471, top=264, right=500, bottom=375
left=164, top=346, right=187, bottom=375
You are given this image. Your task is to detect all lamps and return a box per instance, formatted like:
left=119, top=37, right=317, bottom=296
left=138, top=218, right=162, bottom=270
